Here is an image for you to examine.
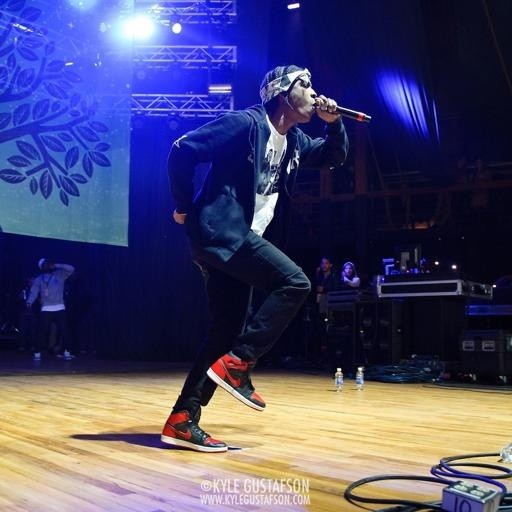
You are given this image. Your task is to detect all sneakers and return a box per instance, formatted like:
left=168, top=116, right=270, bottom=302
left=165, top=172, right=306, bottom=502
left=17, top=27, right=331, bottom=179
left=159, top=409, right=228, bottom=453
left=205, top=351, right=267, bottom=412
left=31, top=349, right=74, bottom=361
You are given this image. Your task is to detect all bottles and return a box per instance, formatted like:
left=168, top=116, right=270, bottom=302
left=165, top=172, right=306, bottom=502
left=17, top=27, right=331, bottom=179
left=355, top=367, right=365, bottom=391
left=334, top=368, right=344, bottom=392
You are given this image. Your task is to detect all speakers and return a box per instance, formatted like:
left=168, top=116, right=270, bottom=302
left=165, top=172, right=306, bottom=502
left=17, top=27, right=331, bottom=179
left=327, top=297, right=512, bottom=384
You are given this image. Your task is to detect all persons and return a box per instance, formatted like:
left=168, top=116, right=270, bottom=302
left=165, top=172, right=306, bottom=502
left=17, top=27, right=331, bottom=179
left=24, top=257, right=79, bottom=361
left=310, top=255, right=340, bottom=369
left=158, top=65, right=348, bottom=454
left=340, top=261, right=359, bottom=289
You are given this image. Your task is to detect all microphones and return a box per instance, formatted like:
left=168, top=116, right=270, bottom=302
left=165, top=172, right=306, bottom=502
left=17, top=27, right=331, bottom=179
left=316, top=101, right=372, bottom=124
